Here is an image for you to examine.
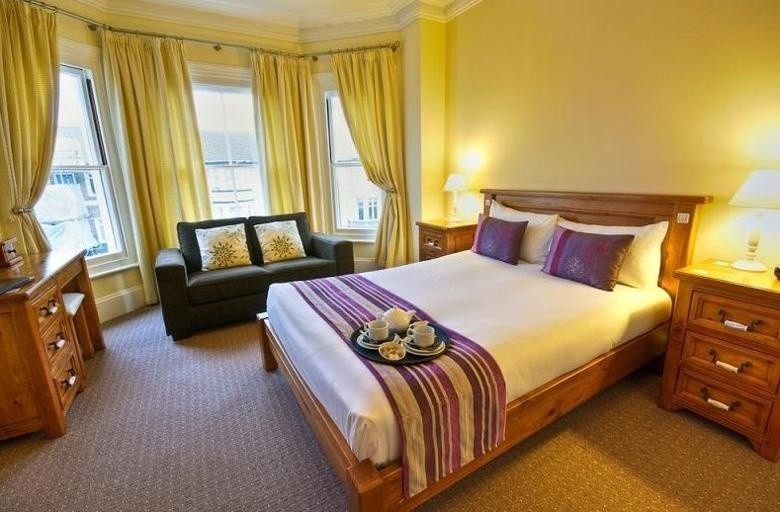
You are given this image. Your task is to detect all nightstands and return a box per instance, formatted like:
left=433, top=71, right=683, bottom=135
left=658, top=257, right=780, bottom=463
left=416, top=219, right=477, bottom=262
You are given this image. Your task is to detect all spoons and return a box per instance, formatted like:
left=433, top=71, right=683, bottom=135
left=393, top=331, right=415, bottom=346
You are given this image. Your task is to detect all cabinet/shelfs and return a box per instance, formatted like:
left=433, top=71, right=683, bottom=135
left=31, top=284, right=79, bottom=410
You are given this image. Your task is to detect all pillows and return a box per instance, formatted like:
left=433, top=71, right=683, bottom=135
left=471, top=212, right=529, bottom=266
left=253, top=220, right=307, bottom=265
left=194, top=223, right=253, bottom=271
left=488, top=199, right=558, bottom=264
left=548, top=216, right=668, bottom=292
left=541, top=224, right=634, bottom=292
left=176, top=217, right=256, bottom=272
left=249, top=212, right=313, bottom=264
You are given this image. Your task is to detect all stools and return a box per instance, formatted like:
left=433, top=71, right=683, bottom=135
left=61, top=293, right=93, bottom=379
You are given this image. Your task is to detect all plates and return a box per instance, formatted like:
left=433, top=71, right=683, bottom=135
left=360, top=331, right=397, bottom=347
left=378, top=341, right=407, bottom=361
left=401, top=336, right=445, bottom=353
left=398, top=337, right=446, bottom=357
left=356, top=334, right=400, bottom=351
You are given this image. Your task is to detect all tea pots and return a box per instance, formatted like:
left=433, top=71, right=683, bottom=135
left=377, top=305, right=416, bottom=333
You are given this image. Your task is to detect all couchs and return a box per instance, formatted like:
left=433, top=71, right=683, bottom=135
left=153, top=232, right=354, bottom=341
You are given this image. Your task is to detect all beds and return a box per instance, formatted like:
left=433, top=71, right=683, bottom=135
left=257, top=188, right=713, bottom=512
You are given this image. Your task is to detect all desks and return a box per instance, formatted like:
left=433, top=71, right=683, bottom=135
left=0, top=249, right=106, bottom=439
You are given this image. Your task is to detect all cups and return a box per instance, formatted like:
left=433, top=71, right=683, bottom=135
left=408, top=319, right=430, bottom=328
left=406, top=325, right=438, bottom=347
left=363, top=320, right=390, bottom=341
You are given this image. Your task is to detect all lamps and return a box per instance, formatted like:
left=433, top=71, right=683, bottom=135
left=442, top=173, right=465, bottom=221
left=727, top=170, right=780, bottom=272
